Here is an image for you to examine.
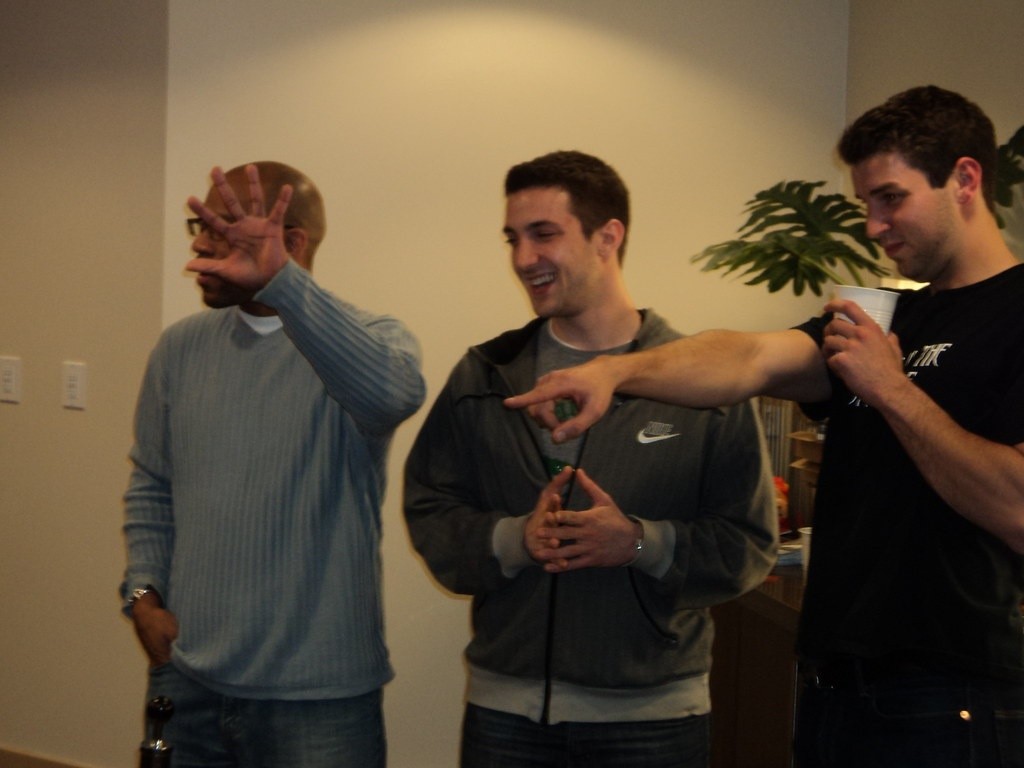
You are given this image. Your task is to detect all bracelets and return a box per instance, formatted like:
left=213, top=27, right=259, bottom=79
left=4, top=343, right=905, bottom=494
left=635, top=531, right=644, bottom=551
left=127, top=588, right=155, bottom=612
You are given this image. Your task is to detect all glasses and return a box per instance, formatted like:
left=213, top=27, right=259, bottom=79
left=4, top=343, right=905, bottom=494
left=186, top=215, right=297, bottom=243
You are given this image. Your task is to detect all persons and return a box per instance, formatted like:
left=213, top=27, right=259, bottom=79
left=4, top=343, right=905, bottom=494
left=117, top=160, right=429, bottom=768
left=404, top=150, right=780, bottom=768
left=504, top=85, right=1024, bottom=768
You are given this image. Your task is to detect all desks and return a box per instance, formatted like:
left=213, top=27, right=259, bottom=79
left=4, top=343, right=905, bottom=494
left=709, top=563, right=807, bottom=768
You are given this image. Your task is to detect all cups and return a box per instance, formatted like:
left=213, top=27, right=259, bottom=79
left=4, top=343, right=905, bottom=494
left=797, top=527, right=812, bottom=586
left=832, top=285, right=901, bottom=338
